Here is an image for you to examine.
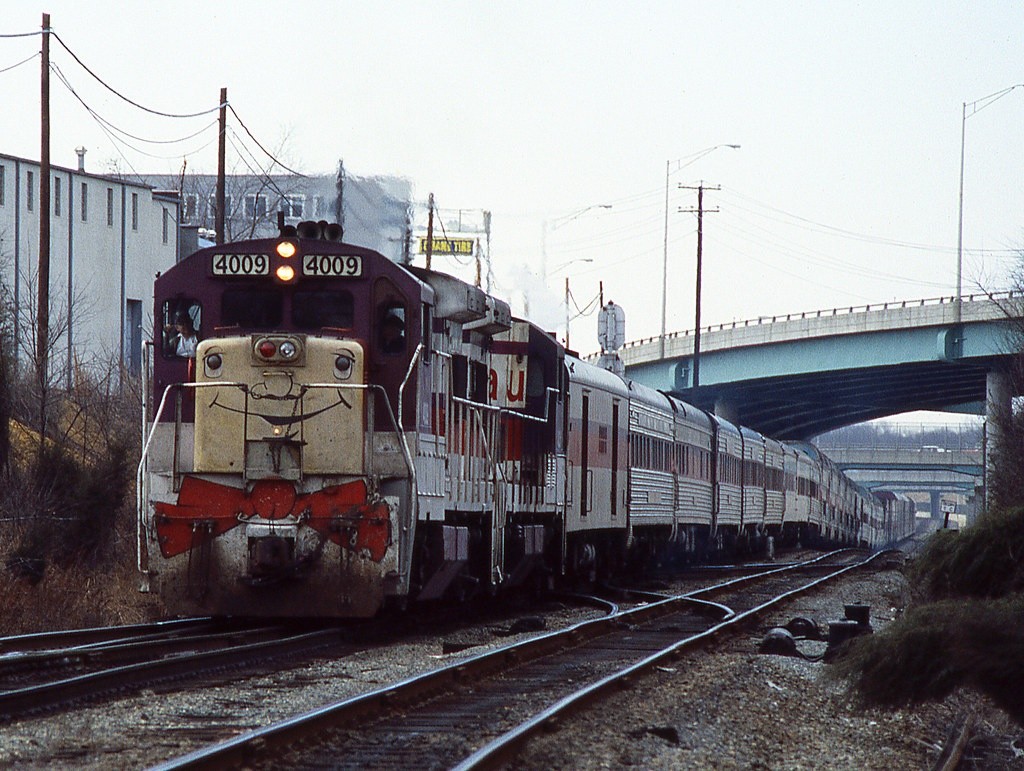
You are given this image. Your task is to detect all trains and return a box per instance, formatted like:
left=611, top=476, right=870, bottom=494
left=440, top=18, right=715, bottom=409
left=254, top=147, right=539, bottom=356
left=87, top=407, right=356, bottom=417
left=136, top=209, right=918, bottom=624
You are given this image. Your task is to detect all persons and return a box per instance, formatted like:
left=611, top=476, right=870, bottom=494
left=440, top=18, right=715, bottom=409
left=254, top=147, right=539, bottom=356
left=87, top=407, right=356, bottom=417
left=170, top=312, right=199, bottom=356
left=382, top=315, right=405, bottom=354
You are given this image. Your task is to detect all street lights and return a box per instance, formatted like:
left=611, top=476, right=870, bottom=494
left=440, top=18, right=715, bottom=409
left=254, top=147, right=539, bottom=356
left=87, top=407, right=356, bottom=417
left=661, top=142, right=743, bottom=336
left=545, top=204, right=611, bottom=328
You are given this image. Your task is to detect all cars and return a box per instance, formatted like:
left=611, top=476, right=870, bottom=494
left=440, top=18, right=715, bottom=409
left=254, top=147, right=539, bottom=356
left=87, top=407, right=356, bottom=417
left=918, top=445, right=950, bottom=452
